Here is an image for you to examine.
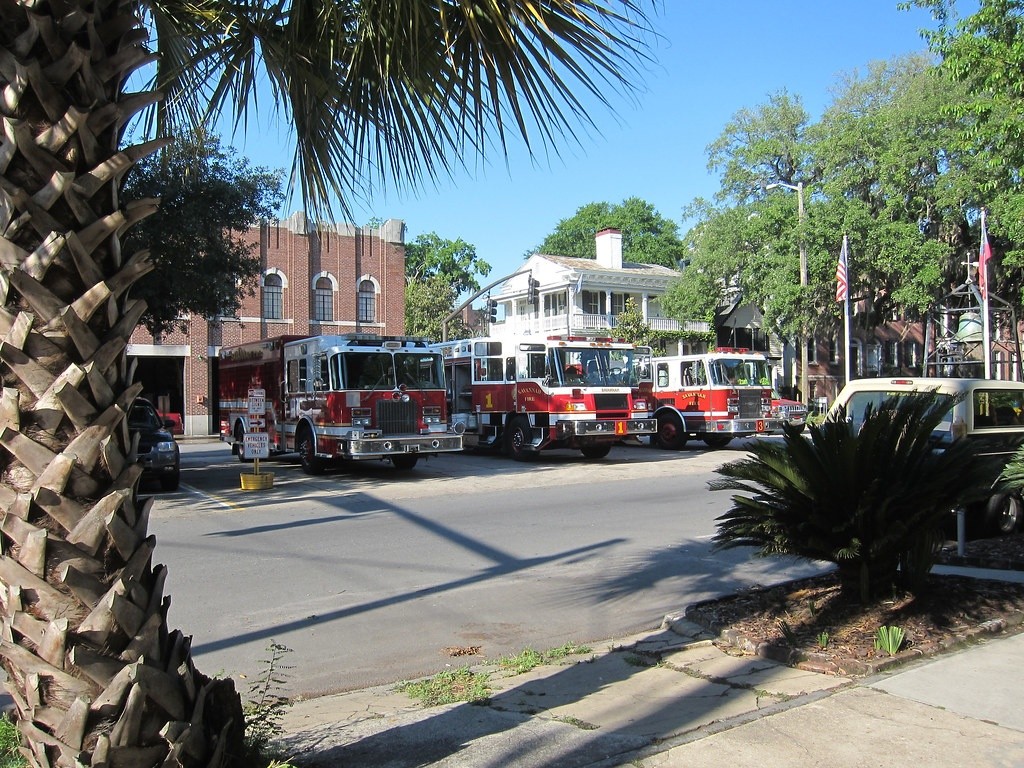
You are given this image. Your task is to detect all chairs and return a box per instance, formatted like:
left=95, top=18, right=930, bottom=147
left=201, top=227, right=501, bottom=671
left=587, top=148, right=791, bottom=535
left=997, top=407, right=1021, bottom=424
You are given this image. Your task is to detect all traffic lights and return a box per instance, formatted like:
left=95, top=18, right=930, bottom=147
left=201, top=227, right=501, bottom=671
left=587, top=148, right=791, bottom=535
left=485, top=301, right=496, bottom=323
left=526, top=279, right=540, bottom=305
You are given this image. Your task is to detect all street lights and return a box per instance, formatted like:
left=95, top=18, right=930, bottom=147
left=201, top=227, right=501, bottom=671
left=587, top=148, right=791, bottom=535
left=766, top=181, right=808, bottom=408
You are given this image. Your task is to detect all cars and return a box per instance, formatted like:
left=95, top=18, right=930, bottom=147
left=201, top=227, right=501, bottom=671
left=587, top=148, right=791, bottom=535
left=127, top=401, right=181, bottom=491
left=770, top=388, right=809, bottom=435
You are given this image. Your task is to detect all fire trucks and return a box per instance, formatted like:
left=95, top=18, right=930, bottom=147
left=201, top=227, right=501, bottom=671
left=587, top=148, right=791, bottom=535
left=422, top=335, right=658, bottom=461
left=612, top=348, right=783, bottom=448
left=218, top=333, right=465, bottom=476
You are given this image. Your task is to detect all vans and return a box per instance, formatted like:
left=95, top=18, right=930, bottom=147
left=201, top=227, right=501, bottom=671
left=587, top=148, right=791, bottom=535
left=820, top=379, right=1024, bottom=534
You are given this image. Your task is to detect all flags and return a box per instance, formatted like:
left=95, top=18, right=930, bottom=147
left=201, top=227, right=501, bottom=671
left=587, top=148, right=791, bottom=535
left=835, top=241, right=849, bottom=302
left=577, top=274, right=583, bottom=293
left=979, top=218, right=992, bottom=293
